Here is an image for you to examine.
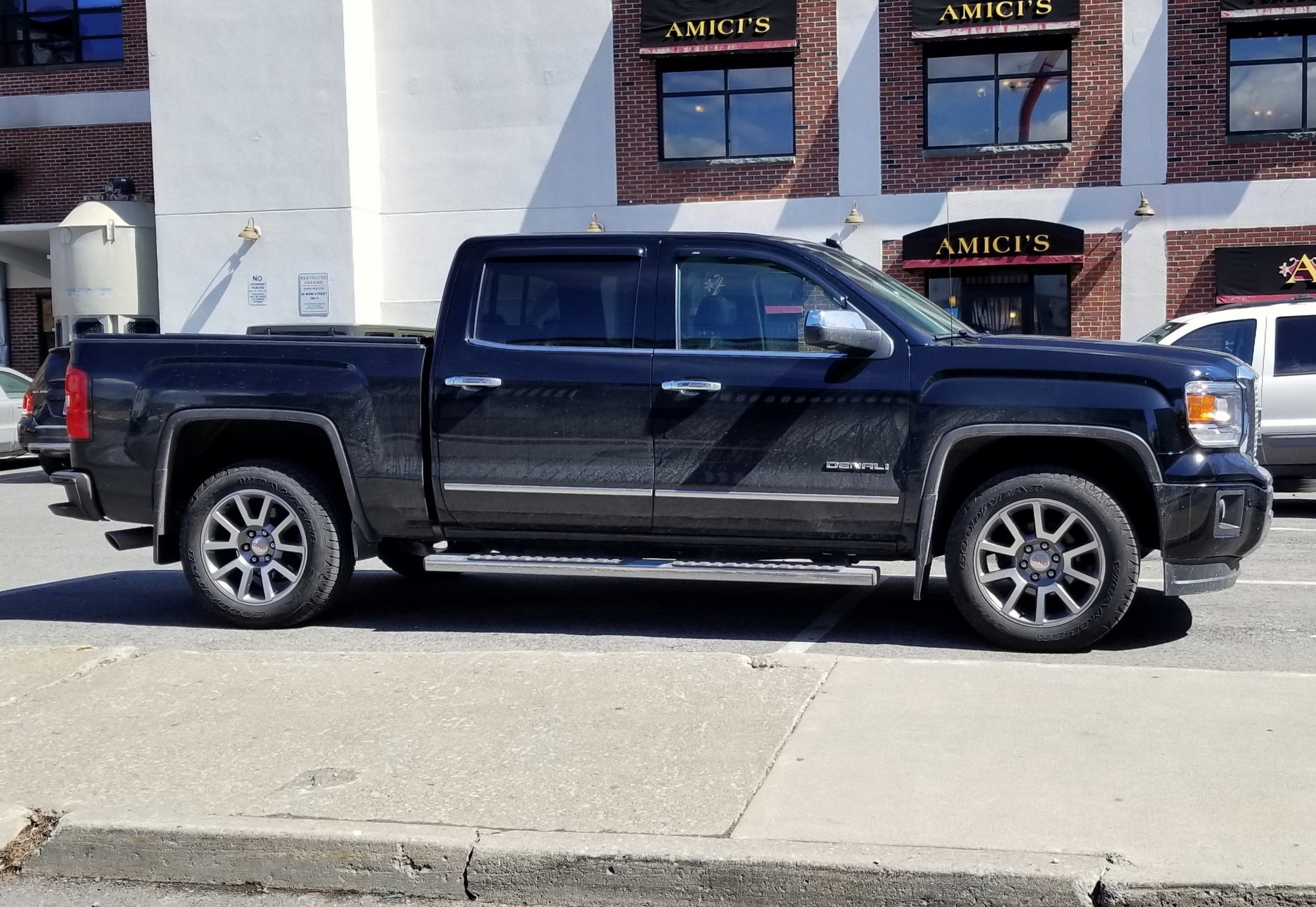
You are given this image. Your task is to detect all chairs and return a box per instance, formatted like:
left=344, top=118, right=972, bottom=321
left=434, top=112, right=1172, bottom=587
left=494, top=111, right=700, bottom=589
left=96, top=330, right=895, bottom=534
left=554, top=285, right=607, bottom=344
left=691, top=296, right=737, bottom=350
left=1232, top=327, right=1249, bottom=363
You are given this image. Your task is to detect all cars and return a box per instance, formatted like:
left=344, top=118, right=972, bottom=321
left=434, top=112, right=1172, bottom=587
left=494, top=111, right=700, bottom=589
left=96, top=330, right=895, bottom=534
left=0, top=367, right=34, bottom=461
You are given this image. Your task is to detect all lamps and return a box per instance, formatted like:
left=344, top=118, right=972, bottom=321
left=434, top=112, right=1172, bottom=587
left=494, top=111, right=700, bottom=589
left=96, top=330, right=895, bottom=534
left=238, top=217, right=262, bottom=242
left=586, top=213, right=604, bottom=232
left=844, top=201, right=864, bottom=228
left=1134, top=190, right=1155, bottom=221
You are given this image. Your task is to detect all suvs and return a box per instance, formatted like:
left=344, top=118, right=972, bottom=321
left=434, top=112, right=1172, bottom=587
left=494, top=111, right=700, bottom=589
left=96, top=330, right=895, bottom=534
left=17, top=340, right=72, bottom=475
left=1138, top=295, right=1316, bottom=468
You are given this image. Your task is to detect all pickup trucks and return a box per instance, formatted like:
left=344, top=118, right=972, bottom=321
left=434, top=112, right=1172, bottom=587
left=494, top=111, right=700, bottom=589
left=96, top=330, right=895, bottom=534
left=51, top=232, right=1275, bottom=651
